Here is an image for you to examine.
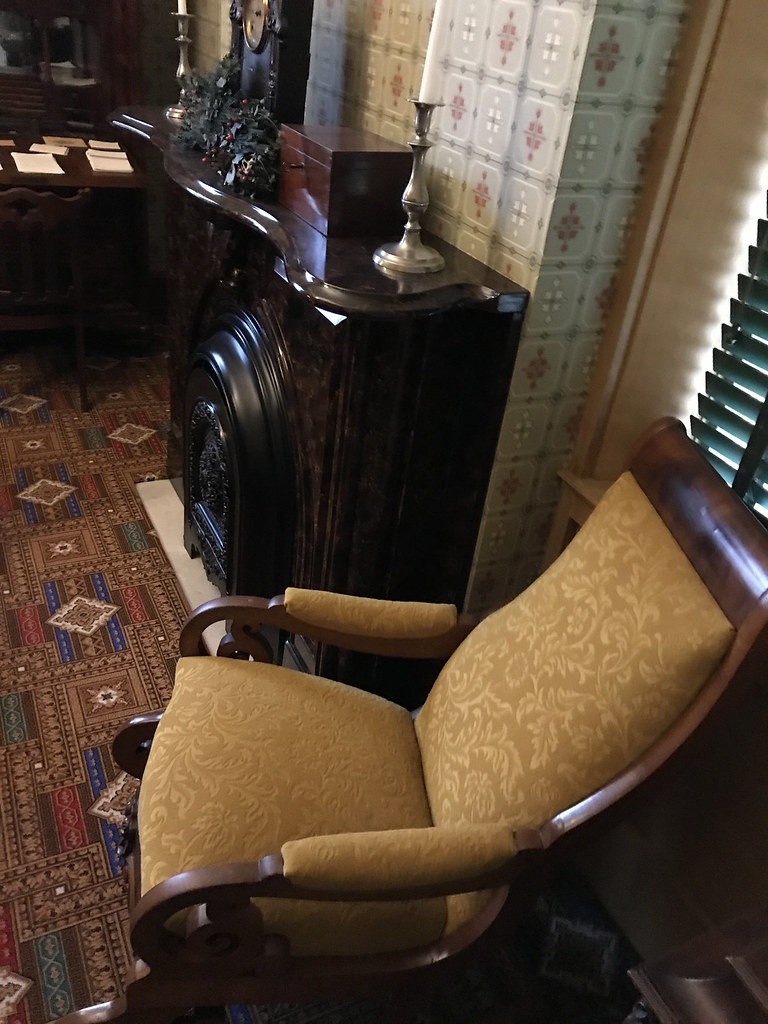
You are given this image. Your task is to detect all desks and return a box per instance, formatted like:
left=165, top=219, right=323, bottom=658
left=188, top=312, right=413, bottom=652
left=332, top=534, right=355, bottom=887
left=0, top=129, right=149, bottom=334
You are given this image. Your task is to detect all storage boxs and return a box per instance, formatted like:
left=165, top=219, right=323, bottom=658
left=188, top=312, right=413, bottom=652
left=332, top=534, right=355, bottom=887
left=276, top=123, right=415, bottom=237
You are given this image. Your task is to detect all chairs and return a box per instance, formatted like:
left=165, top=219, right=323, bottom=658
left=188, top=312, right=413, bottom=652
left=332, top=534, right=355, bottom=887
left=46, top=415, right=768, bottom=1023
left=0, top=188, right=96, bottom=413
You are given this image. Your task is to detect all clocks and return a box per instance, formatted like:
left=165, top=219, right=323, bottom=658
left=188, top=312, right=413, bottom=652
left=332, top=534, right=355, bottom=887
left=228, top=0, right=314, bottom=124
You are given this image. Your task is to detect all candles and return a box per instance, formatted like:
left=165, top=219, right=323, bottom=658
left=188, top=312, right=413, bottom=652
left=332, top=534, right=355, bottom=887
left=178, top=0, right=187, bottom=14
left=418, top=0, right=454, bottom=104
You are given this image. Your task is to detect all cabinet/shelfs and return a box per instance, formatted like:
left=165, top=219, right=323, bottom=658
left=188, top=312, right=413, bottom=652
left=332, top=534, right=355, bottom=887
left=0, top=0, right=141, bottom=139
